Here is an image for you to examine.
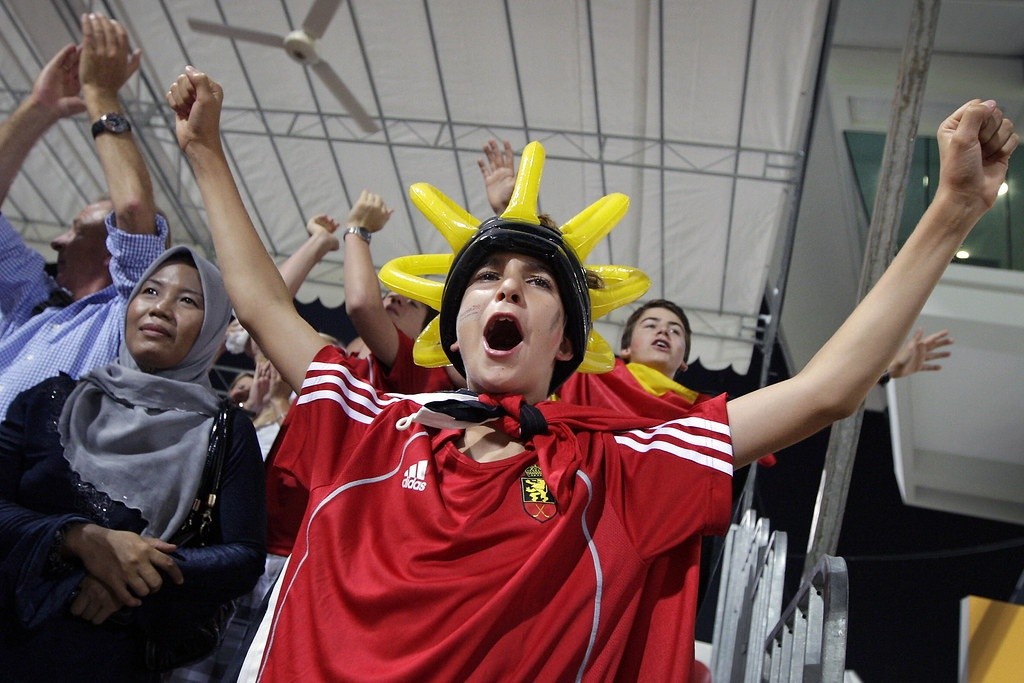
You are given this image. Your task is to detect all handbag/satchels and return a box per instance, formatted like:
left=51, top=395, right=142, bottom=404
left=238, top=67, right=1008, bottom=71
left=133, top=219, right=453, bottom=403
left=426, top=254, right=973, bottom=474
left=145, top=399, right=239, bottom=672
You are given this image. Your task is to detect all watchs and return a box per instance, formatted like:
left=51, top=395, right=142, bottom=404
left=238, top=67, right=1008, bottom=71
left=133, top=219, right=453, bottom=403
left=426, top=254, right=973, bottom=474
left=342, top=226, right=372, bottom=244
left=91, top=112, right=132, bottom=140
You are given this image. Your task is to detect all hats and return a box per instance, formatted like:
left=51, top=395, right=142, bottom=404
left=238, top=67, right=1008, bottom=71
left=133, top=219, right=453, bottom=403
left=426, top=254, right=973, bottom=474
left=377, top=141, right=653, bottom=400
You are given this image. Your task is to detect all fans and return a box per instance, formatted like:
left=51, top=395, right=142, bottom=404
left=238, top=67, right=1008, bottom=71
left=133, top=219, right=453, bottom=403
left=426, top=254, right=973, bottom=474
left=190, top=0, right=379, bottom=133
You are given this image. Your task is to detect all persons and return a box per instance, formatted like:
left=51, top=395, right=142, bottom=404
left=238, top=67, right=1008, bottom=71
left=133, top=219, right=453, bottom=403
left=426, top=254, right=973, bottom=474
left=162, top=65, right=1018, bottom=683
left=0, top=10, right=956, bottom=461
left=0, top=242, right=267, bottom=683
